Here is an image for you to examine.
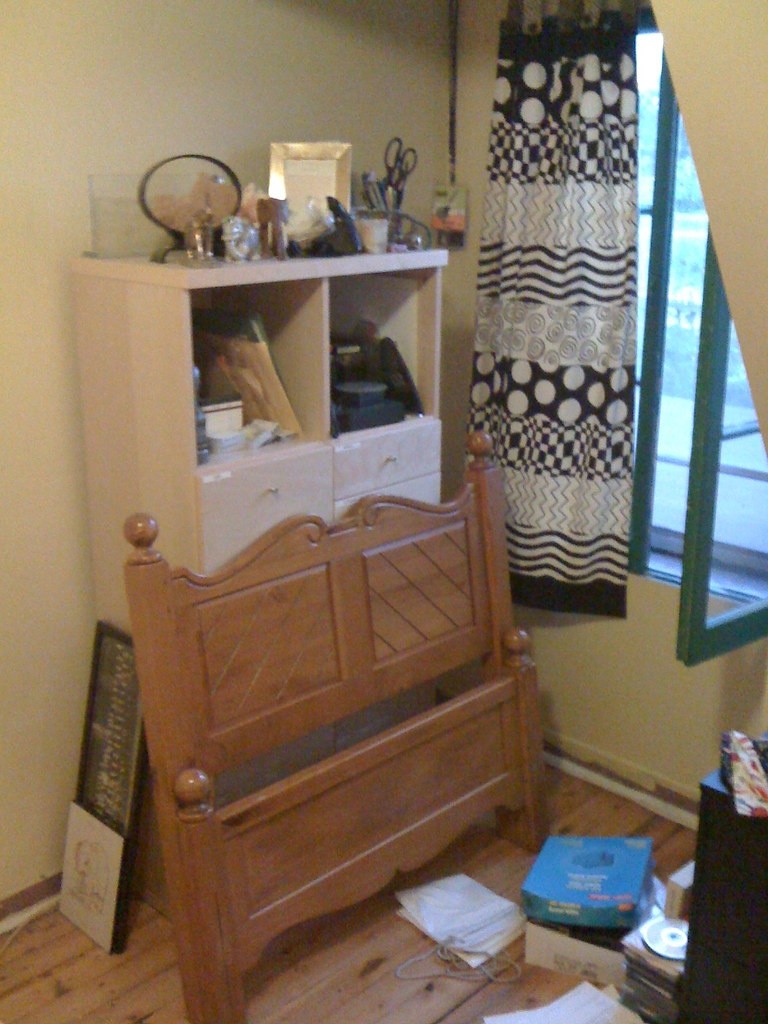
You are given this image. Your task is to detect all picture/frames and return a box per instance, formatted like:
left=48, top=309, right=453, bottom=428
left=271, top=142, right=352, bottom=212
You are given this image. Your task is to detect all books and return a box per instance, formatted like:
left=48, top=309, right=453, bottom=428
left=200, top=393, right=241, bottom=413
left=204, top=304, right=267, bottom=343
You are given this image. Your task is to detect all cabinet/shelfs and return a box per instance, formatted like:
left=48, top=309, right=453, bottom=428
left=73, top=249, right=450, bottom=921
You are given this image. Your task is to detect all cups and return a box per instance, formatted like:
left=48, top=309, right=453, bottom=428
left=354, top=210, right=400, bottom=254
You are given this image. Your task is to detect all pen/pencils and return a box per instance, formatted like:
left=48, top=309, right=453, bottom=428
left=360, top=170, right=405, bottom=212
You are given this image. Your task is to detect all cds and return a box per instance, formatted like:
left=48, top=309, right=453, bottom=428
left=643, top=918, right=689, bottom=960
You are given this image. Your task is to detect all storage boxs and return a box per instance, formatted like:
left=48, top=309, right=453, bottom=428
left=524, top=920, right=627, bottom=988
left=200, top=396, right=243, bottom=436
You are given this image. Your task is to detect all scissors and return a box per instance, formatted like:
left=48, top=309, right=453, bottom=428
left=383, top=136, right=418, bottom=188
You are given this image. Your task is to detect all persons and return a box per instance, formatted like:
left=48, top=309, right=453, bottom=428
left=94, top=642, right=136, bottom=827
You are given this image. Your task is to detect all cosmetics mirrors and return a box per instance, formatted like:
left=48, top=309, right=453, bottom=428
left=137, top=154, right=243, bottom=265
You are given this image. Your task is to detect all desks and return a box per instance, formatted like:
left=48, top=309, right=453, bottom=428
left=678, top=771, right=768, bottom=1024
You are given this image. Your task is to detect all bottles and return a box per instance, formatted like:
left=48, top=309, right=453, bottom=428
left=192, top=363, right=210, bottom=467
